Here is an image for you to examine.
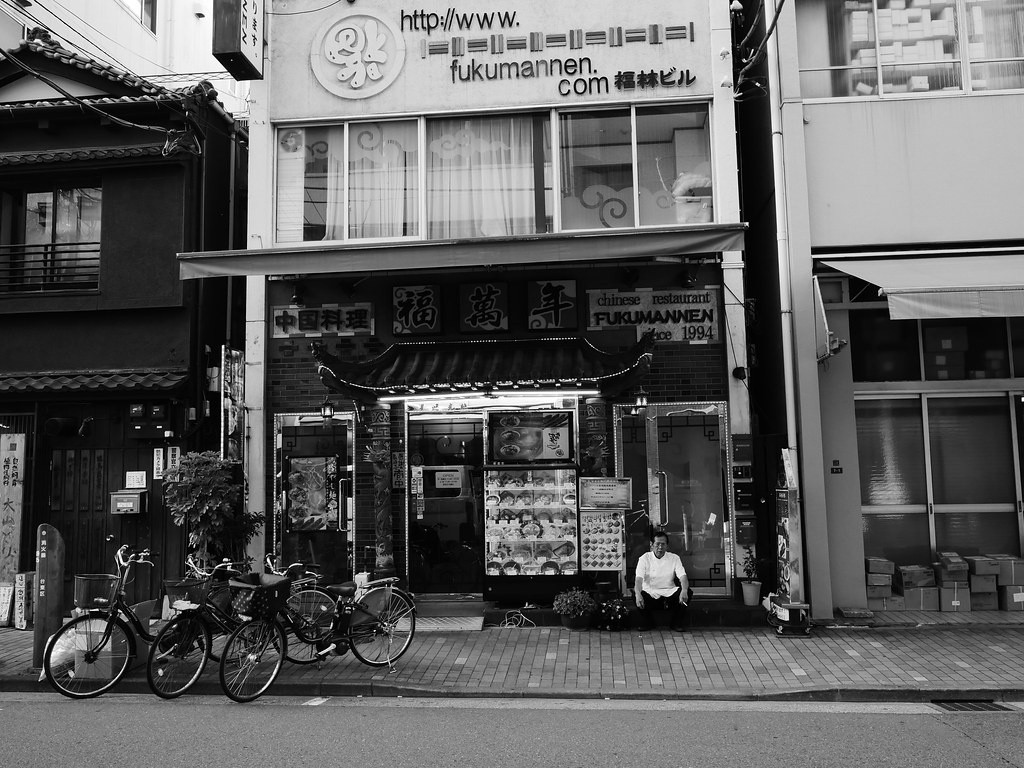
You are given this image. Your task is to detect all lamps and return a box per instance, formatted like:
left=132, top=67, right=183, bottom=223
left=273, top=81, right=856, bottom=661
left=319, top=393, right=335, bottom=418
left=633, top=384, right=649, bottom=408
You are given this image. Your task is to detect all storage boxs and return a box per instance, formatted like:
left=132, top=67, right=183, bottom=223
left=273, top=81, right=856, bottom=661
left=892, top=551, right=1024, bottom=612
left=674, top=196, right=713, bottom=223
left=866, top=585, right=891, bottom=598
left=866, top=572, right=892, bottom=586
left=866, top=556, right=894, bottom=575
left=843, top=0, right=988, bottom=96
left=70, top=597, right=158, bottom=679
left=867, top=594, right=905, bottom=612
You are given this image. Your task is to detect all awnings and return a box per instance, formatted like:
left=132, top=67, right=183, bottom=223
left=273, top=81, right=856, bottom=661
left=820, top=254, right=1024, bottom=320
left=175, top=221, right=746, bottom=281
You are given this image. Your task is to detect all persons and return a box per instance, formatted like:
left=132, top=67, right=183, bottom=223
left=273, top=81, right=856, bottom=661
left=635, top=531, right=693, bottom=632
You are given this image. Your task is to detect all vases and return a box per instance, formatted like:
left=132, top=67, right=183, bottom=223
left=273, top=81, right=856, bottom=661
left=607, top=616, right=625, bottom=631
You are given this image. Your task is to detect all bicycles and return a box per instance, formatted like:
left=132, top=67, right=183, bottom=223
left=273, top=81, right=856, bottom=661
left=43, top=543, right=258, bottom=700
left=218, top=556, right=417, bottom=702
left=141, top=553, right=339, bottom=700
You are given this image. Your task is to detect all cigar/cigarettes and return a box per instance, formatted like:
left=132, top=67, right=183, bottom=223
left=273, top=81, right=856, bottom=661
left=683, top=602, right=688, bottom=606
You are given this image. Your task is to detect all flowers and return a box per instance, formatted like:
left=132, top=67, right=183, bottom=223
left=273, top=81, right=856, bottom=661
left=601, top=598, right=629, bottom=620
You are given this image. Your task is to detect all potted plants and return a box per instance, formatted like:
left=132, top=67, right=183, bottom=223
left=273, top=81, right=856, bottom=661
left=552, top=586, right=598, bottom=630
left=740, top=544, right=766, bottom=606
left=161, top=450, right=268, bottom=633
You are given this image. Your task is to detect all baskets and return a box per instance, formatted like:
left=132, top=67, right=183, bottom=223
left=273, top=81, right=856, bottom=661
left=165, top=577, right=208, bottom=608
left=75, top=574, right=117, bottom=611
left=229, top=572, right=288, bottom=617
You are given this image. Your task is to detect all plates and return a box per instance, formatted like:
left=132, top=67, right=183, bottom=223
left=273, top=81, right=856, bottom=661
left=500, top=430, right=520, bottom=441
left=486, top=475, right=577, bottom=575
left=500, top=445, right=520, bottom=456
left=500, top=417, right=520, bottom=426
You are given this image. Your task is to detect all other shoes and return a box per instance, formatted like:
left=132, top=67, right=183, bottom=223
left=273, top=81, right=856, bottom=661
left=638, top=626, right=651, bottom=631
left=671, top=623, right=685, bottom=633
